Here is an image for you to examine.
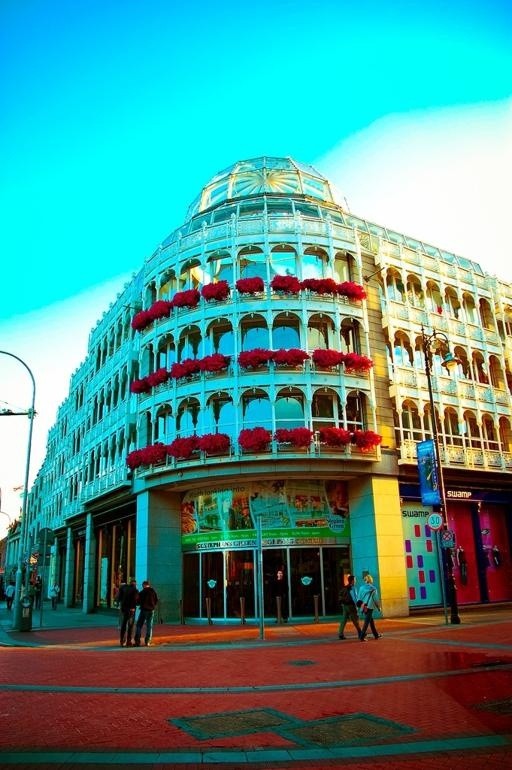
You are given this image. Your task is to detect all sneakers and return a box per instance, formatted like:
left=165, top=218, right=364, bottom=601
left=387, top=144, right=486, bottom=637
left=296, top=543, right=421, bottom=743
left=338, top=633, right=383, bottom=641
left=121, top=643, right=151, bottom=647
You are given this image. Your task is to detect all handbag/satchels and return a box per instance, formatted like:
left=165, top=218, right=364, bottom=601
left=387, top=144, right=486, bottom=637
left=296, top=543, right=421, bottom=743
left=356, top=599, right=368, bottom=617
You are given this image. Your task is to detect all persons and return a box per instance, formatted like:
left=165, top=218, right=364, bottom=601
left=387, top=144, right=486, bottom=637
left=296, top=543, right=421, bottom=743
left=335, top=481, right=347, bottom=515
left=48, top=581, right=61, bottom=610
left=272, top=569, right=289, bottom=625
left=457, top=546, right=467, bottom=584
left=337, top=575, right=362, bottom=640
left=493, top=545, right=501, bottom=567
left=5, top=582, right=14, bottom=609
left=35, top=576, right=41, bottom=608
left=356, top=575, right=383, bottom=642
left=132, top=581, right=158, bottom=647
left=115, top=582, right=138, bottom=647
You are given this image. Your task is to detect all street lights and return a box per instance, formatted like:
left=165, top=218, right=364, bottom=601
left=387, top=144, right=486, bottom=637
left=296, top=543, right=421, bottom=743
left=422, top=332, right=465, bottom=626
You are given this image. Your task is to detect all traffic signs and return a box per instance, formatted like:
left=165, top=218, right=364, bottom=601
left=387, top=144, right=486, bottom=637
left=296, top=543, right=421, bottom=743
left=426, top=512, right=444, bottom=531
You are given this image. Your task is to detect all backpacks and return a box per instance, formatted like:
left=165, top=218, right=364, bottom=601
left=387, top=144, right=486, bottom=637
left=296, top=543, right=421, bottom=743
left=337, top=584, right=352, bottom=605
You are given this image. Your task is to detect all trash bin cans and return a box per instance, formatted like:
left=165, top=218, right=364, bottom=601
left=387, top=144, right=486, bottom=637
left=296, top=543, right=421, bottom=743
left=17, top=596, right=33, bottom=632
